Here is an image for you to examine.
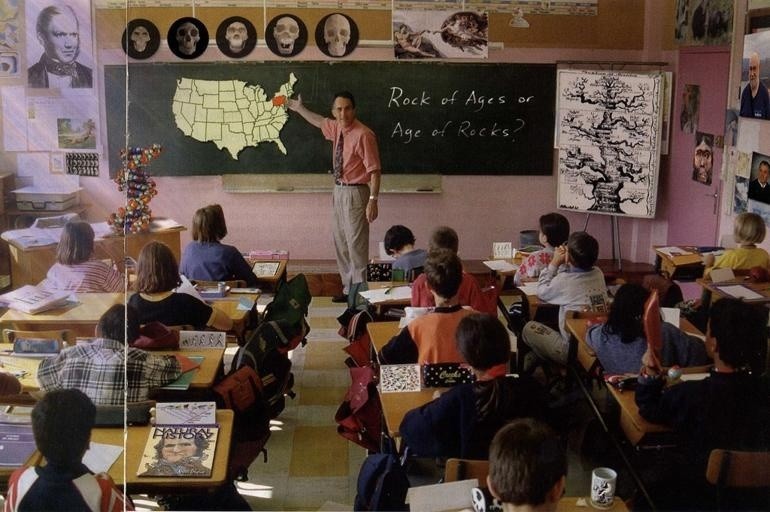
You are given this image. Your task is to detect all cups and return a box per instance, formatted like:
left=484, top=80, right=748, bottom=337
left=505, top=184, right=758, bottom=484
left=588, top=466, right=618, bottom=510
left=217, top=282, right=226, bottom=293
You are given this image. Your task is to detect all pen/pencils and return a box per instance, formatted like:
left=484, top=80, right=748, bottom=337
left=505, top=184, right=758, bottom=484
left=193, top=283, right=199, bottom=288
left=385, top=287, right=394, bottom=294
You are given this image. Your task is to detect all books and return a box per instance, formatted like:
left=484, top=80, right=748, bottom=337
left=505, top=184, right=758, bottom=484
left=1, top=285, right=72, bottom=315
left=136, top=423, right=219, bottom=478
left=0, top=411, right=40, bottom=467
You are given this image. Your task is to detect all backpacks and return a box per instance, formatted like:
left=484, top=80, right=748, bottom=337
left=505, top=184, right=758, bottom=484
left=268, top=273, right=312, bottom=347
left=342, top=310, right=375, bottom=368
left=638, top=270, right=684, bottom=311
left=334, top=362, right=382, bottom=452
left=353, top=445, right=408, bottom=511
left=235, top=319, right=293, bottom=420
left=208, top=363, right=274, bottom=482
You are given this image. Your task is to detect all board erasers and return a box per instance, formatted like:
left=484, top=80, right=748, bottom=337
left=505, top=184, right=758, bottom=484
left=276, top=186, right=295, bottom=191
left=416, top=187, right=433, bottom=191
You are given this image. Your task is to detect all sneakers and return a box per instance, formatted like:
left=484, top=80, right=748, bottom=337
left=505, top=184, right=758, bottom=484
left=331, top=294, right=349, bottom=303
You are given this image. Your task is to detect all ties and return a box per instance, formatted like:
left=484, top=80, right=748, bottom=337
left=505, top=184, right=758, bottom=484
left=332, top=128, right=345, bottom=181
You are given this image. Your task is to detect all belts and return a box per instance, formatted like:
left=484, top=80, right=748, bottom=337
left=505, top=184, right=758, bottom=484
left=334, top=181, right=367, bottom=188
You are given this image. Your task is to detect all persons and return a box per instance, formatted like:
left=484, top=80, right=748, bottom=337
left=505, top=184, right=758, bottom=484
left=3, top=389, right=136, bottom=512
left=128, top=240, right=233, bottom=336
left=179, top=203, right=258, bottom=287
left=378, top=212, right=770, bottom=511
left=37, top=303, right=184, bottom=405
left=46, top=220, right=125, bottom=294
left=0, top=370, right=22, bottom=404
left=26, top=4, right=92, bottom=88
left=748, top=161, right=770, bottom=205
left=738, top=54, right=770, bottom=119
left=283, top=94, right=383, bottom=301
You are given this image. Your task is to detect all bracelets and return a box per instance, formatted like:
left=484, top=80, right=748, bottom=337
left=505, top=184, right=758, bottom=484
left=369, top=196, right=378, bottom=202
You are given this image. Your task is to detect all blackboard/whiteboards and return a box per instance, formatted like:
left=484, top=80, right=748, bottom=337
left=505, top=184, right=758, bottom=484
left=104, top=62, right=558, bottom=180
